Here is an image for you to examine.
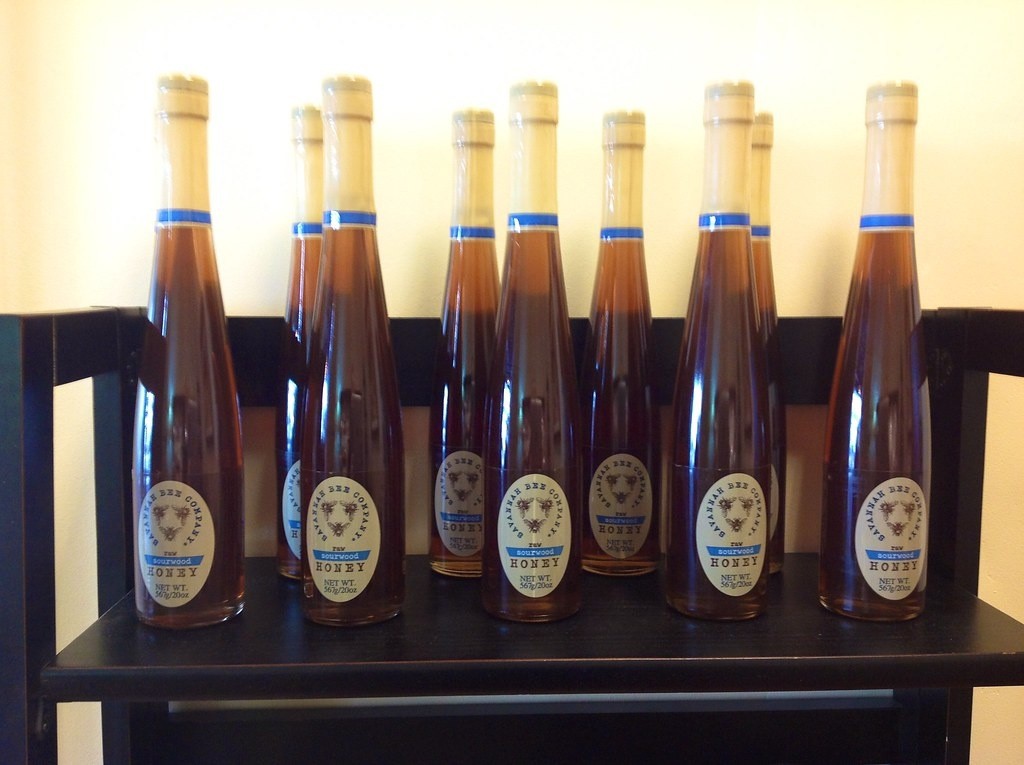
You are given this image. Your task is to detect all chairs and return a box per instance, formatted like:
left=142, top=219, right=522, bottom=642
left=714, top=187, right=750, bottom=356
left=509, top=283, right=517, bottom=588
left=0, top=304, right=1024, bottom=765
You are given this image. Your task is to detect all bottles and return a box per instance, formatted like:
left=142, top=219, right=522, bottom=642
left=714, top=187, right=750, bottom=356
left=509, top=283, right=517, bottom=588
left=133, top=71, right=933, bottom=631
